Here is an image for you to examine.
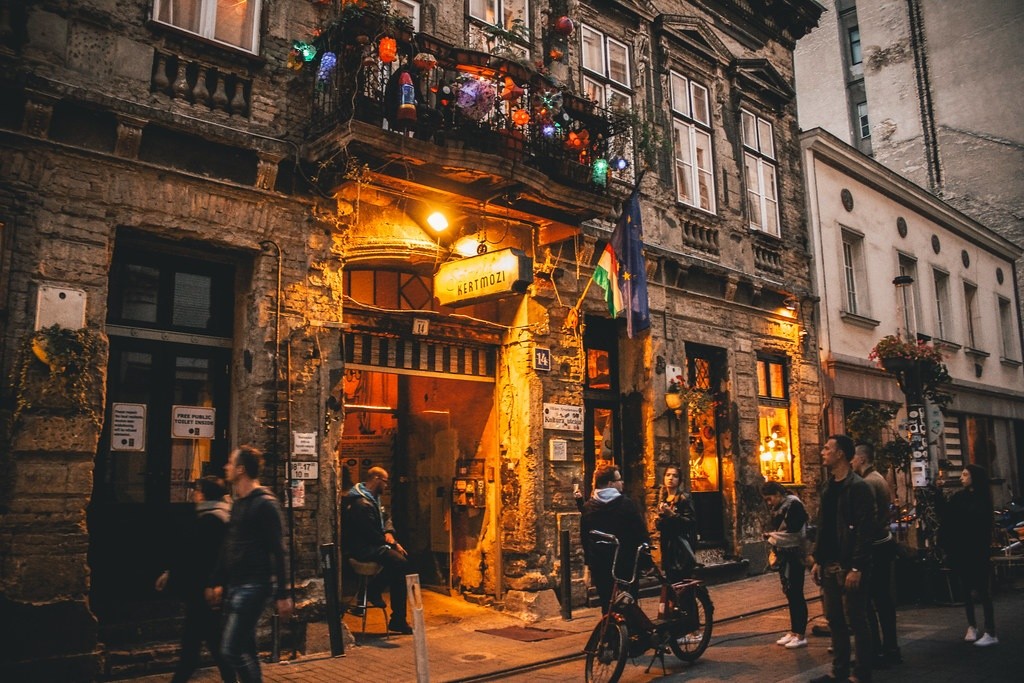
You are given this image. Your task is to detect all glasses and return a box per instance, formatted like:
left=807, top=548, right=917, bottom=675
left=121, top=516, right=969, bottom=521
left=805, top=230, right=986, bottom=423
left=611, top=476, right=622, bottom=482
left=377, top=477, right=388, bottom=483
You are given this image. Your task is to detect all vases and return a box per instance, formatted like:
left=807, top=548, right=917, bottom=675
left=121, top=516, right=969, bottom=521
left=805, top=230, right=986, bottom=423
left=883, top=357, right=907, bottom=370
left=665, top=393, right=682, bottom=409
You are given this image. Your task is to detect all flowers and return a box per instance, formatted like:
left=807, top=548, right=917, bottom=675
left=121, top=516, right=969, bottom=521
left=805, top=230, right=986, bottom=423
left=666, top=375, right=709, bottom=407
left=869, top=334, right=943, bottom=367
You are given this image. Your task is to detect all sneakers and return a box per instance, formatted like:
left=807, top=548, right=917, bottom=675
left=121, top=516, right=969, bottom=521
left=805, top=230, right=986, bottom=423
left=785, top=637, right=807, bottom=649
left=776, top=634, right=796, bottom=645
left=964, top=626, right=978, bottom=640
left=975, top=632, right=998, bottom=646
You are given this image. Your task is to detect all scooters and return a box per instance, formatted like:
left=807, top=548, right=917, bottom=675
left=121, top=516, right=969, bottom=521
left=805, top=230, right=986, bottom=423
left=580, top=531, right=716, bottom=683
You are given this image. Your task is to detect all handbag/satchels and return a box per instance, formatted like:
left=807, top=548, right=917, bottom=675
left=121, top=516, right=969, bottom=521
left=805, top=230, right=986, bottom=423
left=662, top=532, right=696, bottom=574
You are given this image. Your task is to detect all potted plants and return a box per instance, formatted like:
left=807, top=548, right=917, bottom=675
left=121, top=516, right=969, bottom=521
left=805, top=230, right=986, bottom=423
left=6, top=322, right=108, bottom=443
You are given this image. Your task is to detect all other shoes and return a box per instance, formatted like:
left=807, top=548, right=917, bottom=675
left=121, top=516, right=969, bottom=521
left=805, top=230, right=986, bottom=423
left=677, top=634, right=702, bottom=644
left=361, top=589, right=386, bottom=608
left=348, top=606, right=365, bottom=617
left=388, top=621, right=413, bottom=635
left=810, top=620, right=904, bottom=683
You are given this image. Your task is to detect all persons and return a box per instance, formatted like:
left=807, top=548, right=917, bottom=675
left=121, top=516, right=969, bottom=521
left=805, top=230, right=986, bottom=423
left=940, top=463, right=999, bottom=646
left=580, top=464, right=651, bottom=616
left=806, top=430, right=904, bottom=682
left=757, top=481, right=810, bottom=649
left=655, top=465, right=702, bottom=643
left=211, top=445, right=294, bottom=683
left=156, top=474, right=238, bottom=683
left=347, top=465, right=414, bottom=633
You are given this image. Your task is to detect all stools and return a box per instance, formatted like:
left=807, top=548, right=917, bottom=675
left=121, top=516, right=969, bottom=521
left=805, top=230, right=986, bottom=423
left=345, top=560, right=389, bottom=643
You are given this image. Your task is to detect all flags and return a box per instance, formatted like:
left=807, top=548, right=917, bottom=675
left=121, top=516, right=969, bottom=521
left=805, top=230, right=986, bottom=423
left=591, top=188, right=650, bottom=339
